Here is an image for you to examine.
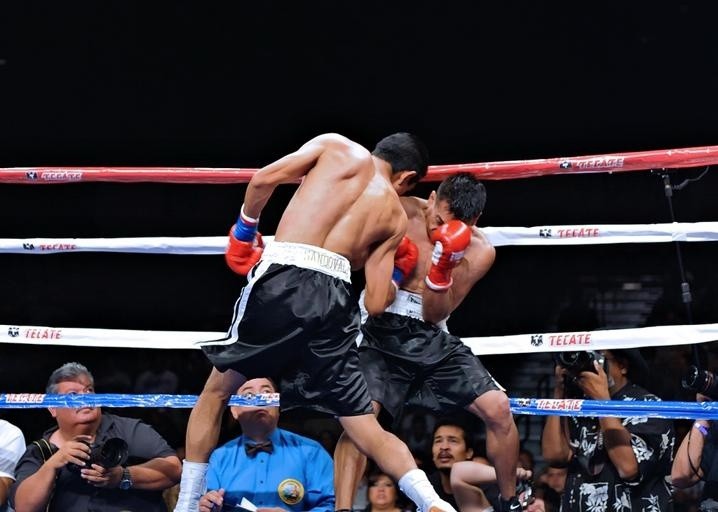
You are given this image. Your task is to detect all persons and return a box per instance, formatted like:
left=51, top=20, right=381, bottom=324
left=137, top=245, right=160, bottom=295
left=0, top=324, right=718, bottom=512
left=332, top=172, right=522, bottom=512
left=171, top=131, right=456, bottom=512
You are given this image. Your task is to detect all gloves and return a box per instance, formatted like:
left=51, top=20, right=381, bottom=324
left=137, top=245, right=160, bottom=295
left=227, top=204, right=264, bottom=275
left=392, top=237, right=419, bottom=292
left=425, top=221, right=471, bottom=292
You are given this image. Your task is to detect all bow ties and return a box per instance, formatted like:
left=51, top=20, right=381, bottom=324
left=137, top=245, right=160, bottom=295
left=244, top=441, right=272, bottom=456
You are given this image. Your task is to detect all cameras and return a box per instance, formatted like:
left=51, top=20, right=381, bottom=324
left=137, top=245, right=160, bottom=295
left=681, top=365, right=718, bottom=401
left=72, top=436, right=129, bottom=469
left=557, top=350, right=607, bottom=382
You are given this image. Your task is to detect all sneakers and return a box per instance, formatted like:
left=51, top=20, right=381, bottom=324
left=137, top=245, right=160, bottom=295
left=421, top=499, right=457, bottom=512
left=498, top=494, right=523, bottom=512
